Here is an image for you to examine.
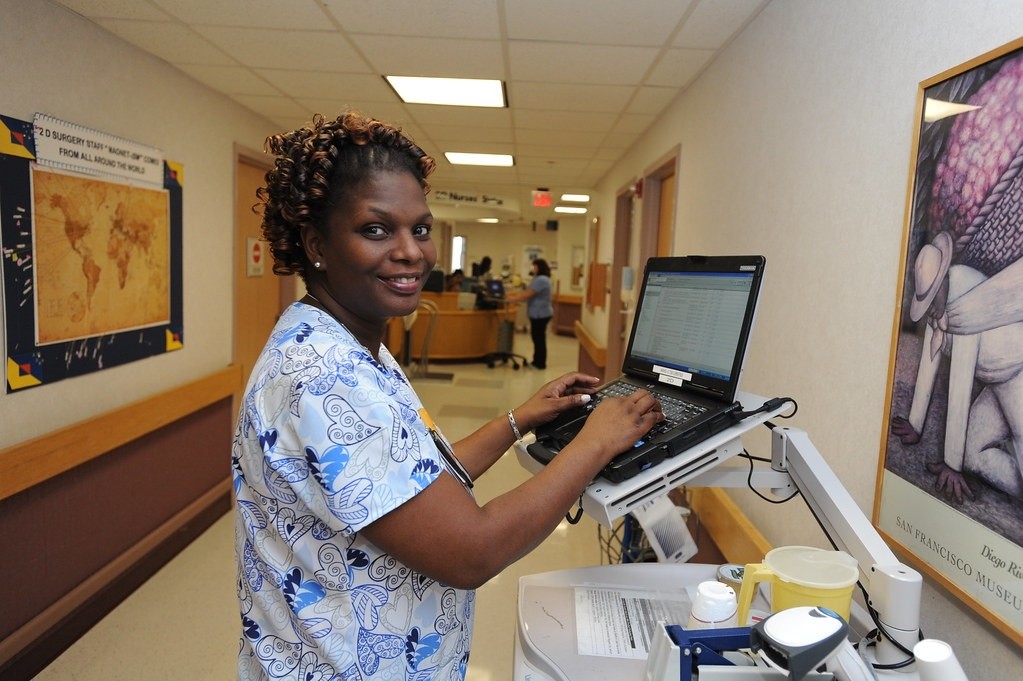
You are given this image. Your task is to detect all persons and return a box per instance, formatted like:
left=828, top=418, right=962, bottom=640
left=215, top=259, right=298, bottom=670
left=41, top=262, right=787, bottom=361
left=508, top=259, right=554, bottom=370
left=231, top=108, right=667, bottom=681
left=446, top=269, right=464, bottom=292
left=476, top=256, right=495, bottom=290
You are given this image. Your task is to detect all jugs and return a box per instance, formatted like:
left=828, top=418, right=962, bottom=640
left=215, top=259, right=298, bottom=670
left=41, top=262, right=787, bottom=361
left=737, top=546, right=859, bottom=628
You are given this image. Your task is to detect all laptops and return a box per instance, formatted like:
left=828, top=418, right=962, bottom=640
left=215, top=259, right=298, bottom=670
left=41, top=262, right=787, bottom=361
left=486, top=280, right=506, bottom=299
left=532, top=255, right=767, bottom=484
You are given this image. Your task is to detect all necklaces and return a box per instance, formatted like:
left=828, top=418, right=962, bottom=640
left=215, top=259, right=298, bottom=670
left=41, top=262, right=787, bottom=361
left=305, top=293, right=383, bottom=369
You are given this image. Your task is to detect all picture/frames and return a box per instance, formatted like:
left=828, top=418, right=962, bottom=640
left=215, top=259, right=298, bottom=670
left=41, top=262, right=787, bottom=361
left=869, top=38, right=1023, bottom=652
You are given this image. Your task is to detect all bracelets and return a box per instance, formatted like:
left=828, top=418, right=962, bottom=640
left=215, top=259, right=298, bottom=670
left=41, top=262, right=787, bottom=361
left=507, top=408, right=524, bottom=440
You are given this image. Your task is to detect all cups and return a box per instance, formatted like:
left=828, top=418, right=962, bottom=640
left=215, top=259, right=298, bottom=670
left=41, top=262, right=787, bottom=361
left=688, top=581, right=738, bottom=630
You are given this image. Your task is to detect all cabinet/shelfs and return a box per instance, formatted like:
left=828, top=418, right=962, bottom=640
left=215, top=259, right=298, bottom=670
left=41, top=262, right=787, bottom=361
left=514, top=563, right=926, bottom=681
